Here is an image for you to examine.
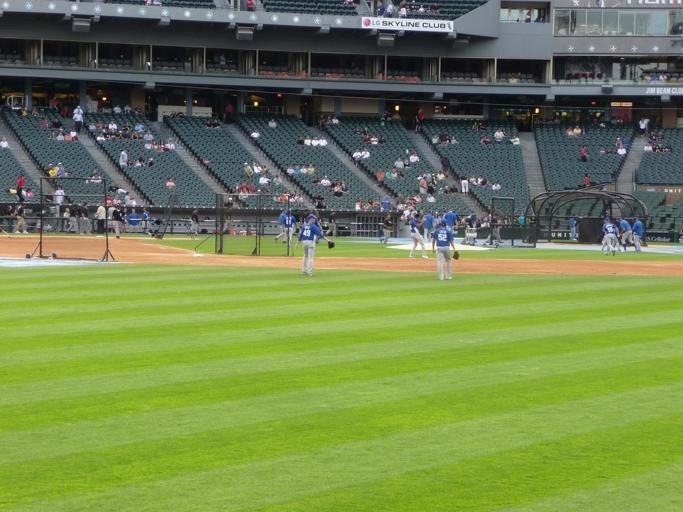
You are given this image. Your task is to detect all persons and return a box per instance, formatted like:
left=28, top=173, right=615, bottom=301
left=584, top=174, right=592, bottom=188
left=565, top=116, right=670, bottom=163
left=251, top=118, right=277, bottom=139
left=10, top=162, right=176, bottom=238
left=296, top=216, right=331, bottom=277
left=1, top=94, right=86, bottom=147
left=234, top=161, right=314, bottom=206
left=89, top=103, right=184, bottom=169
left=431, top=121, right=524, bottom=146
left=568, top=217, right=645, bottom=257
left=204, top=104, right=234, bottom=129
left=432, top=220, right=458, bottom=280
left=643, top=71, right=667, bottom=82
left=246, top=1, right=257, bottom=11
left=214, top=53, right=228, bottom=68
left=508, top=73, right=522, bottom=84
left=356, top=111, right=401, bottom=144
left=312, top=175, right=348, bottom=213
left=408, top=211, right=430, bottom=259
left=375, top=1, right=440, bottom=19
left=298, top=113, right=339, bottom=147
left=352, top=150, right=501, bottom=246
left=413, top=108, right=425, bottom=134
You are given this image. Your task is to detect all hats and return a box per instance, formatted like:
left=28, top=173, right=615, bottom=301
left=280, top=207, right=319, bottom=223
left=441, top=219, right=447, bottom=224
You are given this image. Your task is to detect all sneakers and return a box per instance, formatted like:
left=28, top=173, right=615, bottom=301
left=440, top=276, right=452, bottom=280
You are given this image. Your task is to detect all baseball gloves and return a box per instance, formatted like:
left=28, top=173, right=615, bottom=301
left=328, top=241, right=334, bottom=248
left=453, top=251, right=459, bottom=259
